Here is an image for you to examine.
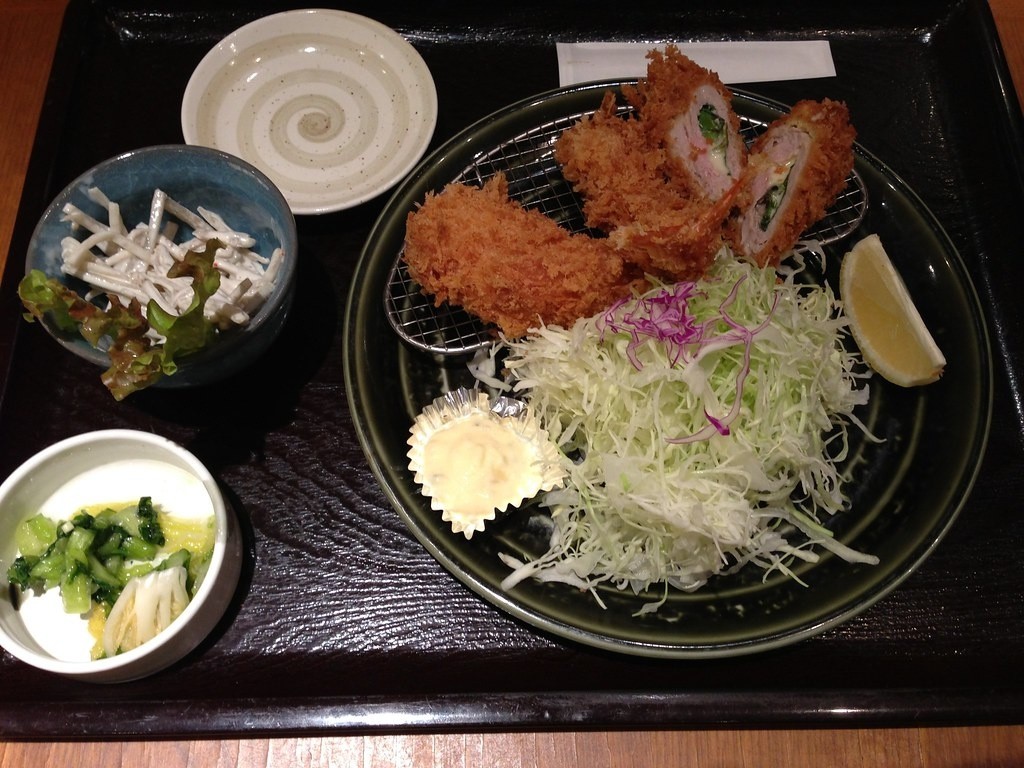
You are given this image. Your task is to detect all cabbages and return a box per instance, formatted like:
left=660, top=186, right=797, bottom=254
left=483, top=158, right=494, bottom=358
left=501, top=251, right=882, bottom=607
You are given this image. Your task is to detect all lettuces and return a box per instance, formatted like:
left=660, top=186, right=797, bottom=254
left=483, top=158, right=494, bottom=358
left=14, top=244, right=232, bottom=400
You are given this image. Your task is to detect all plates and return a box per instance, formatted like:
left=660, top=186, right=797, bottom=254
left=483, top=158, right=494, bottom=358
left=179, top=9, right=437, bottom=217
left=343, top=74, right=994, bottom=664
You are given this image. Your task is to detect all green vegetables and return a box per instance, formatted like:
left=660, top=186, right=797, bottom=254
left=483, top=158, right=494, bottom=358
left=11, top=496, right=214, bottom=612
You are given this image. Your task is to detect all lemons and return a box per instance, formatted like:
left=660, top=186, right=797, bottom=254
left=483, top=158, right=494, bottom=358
left=840, top=232, right=946, bottom=388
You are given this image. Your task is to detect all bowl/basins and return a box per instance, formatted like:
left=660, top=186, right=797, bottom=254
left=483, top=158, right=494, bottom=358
left=1, top=427, right=244, bottom=686
left=22, top=142, right=300, bottom=389
